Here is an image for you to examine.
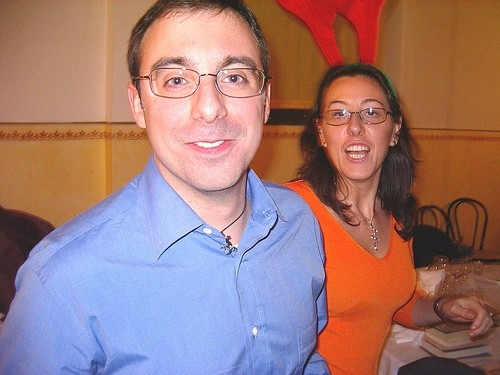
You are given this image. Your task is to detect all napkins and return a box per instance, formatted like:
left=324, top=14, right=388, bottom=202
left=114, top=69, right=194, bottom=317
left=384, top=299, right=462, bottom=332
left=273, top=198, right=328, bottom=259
left=392, top=270, right=445, bottom=347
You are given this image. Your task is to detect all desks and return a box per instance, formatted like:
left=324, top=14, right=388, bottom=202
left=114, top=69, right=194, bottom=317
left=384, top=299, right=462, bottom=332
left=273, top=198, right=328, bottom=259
left=377, top=262, right=500, bottom=375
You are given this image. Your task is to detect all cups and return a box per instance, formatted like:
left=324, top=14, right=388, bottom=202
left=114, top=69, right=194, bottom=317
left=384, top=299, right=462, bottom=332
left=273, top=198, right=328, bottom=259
left=429, top=256, right=484, bottom=285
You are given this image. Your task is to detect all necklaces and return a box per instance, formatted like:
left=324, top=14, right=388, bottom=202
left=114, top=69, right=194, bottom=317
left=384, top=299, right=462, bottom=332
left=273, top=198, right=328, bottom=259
left=220, top=177, right=249, bottom=247
left=339, top=192, right=384, bottom=250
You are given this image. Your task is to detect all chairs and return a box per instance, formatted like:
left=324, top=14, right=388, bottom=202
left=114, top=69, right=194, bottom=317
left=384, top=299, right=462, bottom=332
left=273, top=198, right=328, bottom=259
left=417, top=198, right=500, bottom=260
left=0, top=205, right=55, bottom=322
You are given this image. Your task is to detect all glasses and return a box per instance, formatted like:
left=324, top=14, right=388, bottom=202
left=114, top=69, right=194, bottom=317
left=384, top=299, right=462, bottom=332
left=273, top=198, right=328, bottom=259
left=315, top=107, right=397, bottom=126
left=131, top=67, right=273, bottom=99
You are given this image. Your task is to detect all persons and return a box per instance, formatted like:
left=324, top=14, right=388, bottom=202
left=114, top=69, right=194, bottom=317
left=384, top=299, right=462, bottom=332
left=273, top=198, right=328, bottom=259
left=1, top=1, right=328, bottom=375
left=277, top=63, right=495, bottom=375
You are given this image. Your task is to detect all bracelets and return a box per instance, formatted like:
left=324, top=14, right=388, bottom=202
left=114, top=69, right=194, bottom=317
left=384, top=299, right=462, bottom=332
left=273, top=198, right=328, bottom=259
left=433, top=294, right=451, bottom=323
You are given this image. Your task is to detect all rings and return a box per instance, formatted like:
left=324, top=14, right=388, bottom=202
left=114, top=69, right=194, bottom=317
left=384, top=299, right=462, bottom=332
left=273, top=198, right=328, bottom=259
left=489, top=312, right=494, bottom=317
